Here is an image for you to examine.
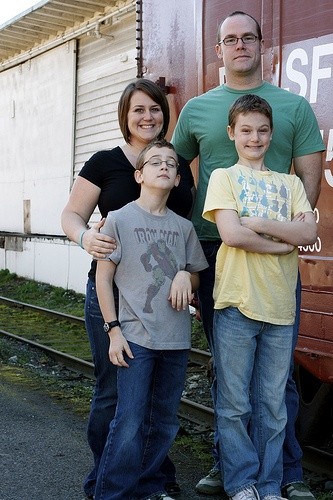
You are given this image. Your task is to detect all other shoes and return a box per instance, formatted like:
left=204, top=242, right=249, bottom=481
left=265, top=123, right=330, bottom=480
left=227, top=486, right=257, bottom=500
left=260, top=495, right=288, bottom=500
left=147, top=493, right=176, bottom=500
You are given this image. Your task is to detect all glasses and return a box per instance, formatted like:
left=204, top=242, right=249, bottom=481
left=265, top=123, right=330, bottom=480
left=217, top=34, right=261, bottom=46
left=138, top=157, right=179, bottom=172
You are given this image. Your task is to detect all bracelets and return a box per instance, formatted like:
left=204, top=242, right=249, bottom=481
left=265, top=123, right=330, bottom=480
left=81, top=230, right=87, bottom=249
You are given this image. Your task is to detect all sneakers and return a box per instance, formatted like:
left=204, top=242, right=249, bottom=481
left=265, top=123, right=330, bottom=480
left=195, top=467, right=225, bottom=495
left=284, top=482, right=316, bottom=500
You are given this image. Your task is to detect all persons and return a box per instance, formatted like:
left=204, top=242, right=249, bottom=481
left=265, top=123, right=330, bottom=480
left=202, top=95, right=318, bottom=500
left=95, top=139, right=209, bottom=500
left=171, top=12, right=315, bottom=500
left=61, top=79, right=196, bottom=500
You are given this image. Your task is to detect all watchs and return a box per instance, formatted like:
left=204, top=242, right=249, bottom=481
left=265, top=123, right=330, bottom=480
left=103, top=320, right=120, bottom=333
